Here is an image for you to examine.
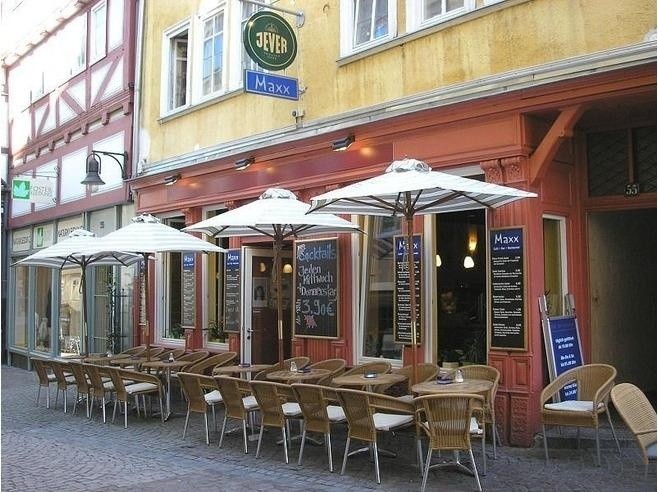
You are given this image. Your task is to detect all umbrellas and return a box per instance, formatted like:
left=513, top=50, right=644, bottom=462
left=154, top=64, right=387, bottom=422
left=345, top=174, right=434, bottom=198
left=8, top=229, right=144, bottom=357
left=304, top=158, right=538, bottom=464
left=45, top=211, right=229, bottom=375
left=179, top=186, right=362, bottom=437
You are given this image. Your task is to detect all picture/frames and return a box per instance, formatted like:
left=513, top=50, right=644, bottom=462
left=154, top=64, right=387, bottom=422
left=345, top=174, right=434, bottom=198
left=252, top=277, right=268, bottom=308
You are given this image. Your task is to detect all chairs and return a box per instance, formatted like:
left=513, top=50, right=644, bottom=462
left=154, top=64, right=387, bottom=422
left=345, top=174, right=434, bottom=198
left=248, top=379, right=305, bottom=465
left=393, top=363, right=438, bottom=394
left=305, top=359, right=348, bottom=386
left=441, top=366, right=501, bottom=462
left=338, top=361, right=392, bottom=392
left=274, top=358, right=311, bottom=384
left=414, top=395, right=485, bottom=492
left=176, top=372, right=215, bottom=445
left=292, top=383, right=346, bottom=472
left=213, top=374, right=260, bottom=454
left=540, top=363, right=622, bottom=467
left=611, top=383, right=656, bottom=480
left=334, top=389, right=416, bottom=484
left=29, top=344, right=237, bottom=429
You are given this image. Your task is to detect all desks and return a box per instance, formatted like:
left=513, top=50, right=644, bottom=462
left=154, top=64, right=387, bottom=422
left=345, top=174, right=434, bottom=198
left=268, top=369, right=332, bottom=445
left=213, top=364, right=272, bottom=434
left=334, top=374, right=407, bottom=458
left=413, top=377, right=493, bottom=478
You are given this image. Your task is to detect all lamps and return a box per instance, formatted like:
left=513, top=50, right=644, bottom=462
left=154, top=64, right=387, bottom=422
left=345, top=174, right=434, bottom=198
left=80, top=150, right=129, bottom=183
left=163, top=173, right=182, bottom=187
left=329, top=133, right=356, bottom=151
left=235, top=157, right=256, bottom=171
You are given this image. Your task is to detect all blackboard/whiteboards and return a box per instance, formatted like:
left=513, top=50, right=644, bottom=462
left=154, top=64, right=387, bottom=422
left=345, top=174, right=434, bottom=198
left=290, top=237, right=341, bottom=341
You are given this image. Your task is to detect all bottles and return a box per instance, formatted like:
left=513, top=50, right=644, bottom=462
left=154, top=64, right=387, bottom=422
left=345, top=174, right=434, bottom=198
left=289, top=361, right=297, bottom=372
left=455, top=369, right=464, bottom=383
left=106, top=348, right=113, bottom=358
left=168, top=352, right=175, bottom=364
